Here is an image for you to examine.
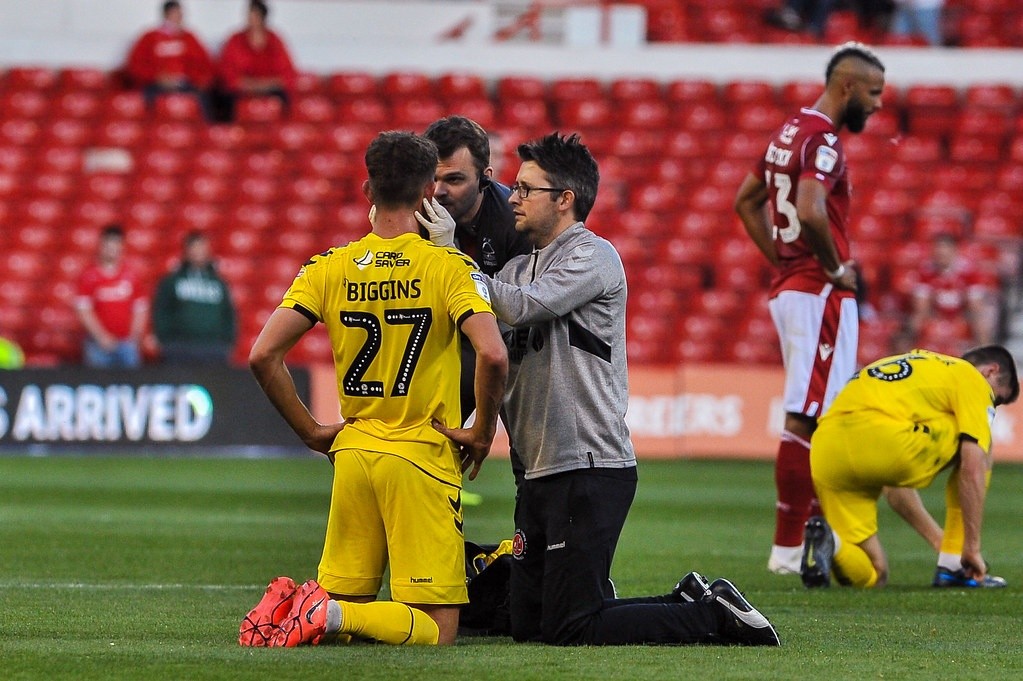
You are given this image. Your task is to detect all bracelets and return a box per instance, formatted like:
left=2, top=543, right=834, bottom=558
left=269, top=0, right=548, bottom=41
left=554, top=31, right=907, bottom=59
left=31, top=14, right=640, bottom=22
left=824, top=264, right=845, bottom=279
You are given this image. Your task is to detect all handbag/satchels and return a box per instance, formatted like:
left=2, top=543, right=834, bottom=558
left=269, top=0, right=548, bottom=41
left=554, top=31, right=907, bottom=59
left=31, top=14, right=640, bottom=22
left=459, top=540, right=516, bottom=637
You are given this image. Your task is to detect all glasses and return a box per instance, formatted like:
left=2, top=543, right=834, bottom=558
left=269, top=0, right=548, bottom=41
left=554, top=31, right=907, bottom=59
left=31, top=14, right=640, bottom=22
left=510, top=185, right=565, bottom=198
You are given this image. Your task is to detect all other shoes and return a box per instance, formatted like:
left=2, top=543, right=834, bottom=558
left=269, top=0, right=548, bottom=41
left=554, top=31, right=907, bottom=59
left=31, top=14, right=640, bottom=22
left=766, top=542, right=806, bottom=575
left=605, top=578, right=617, bottom=599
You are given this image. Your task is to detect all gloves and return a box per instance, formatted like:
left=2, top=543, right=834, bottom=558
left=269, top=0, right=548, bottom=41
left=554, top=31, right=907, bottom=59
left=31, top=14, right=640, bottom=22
left=414, top=197, right=456, bottom=248
left=368, top=204, right=377, bottom=228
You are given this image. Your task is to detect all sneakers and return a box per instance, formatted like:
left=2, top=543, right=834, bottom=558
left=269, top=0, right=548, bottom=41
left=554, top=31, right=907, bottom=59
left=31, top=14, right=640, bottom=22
left=933, top=559, right=1007, bottom=590
left=267, top=579, right=331, bottom=650
left=239, top=577, right=299, bottom=647
left=800, top=516, right=834, bottom=590
left=700, top=577, right=780, bottom=646
left=673, top=572, right=710, bottom=604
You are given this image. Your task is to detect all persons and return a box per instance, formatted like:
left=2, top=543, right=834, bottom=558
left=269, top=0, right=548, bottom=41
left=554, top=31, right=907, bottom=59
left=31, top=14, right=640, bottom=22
left=369, top=130, right=782, bottom=646
left=422, top=117, right=535, bottom=531
left=73, top=223, right=236, bottom=372
left=123, top=0, right=297, bottom=125
left=800, top=345, right=1020, bottom=590
left=733, top=48, right=886, bottom=577
left=762, top=0, right=948, bottom=46
left=237, top=129, right=509, bottom=650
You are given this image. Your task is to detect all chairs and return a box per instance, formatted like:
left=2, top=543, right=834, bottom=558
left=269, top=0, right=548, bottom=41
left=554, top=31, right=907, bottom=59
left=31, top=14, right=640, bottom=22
left=0, top=0, right=1023, bottom=367
